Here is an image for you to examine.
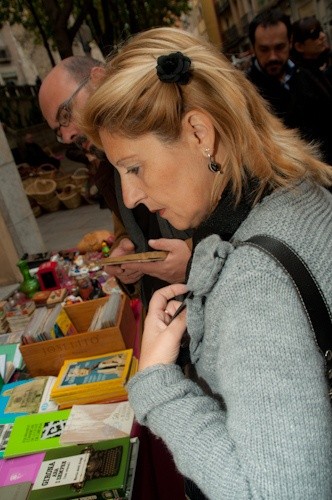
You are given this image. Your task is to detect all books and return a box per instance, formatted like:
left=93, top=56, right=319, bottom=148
left=0, top=284, right=140, bottom=500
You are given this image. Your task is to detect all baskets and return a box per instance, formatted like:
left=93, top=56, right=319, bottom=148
left=17, top=164, right=97, bottom=217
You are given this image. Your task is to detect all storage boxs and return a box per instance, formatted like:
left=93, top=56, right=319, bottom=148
left=18, top=294, right=137, bottom=378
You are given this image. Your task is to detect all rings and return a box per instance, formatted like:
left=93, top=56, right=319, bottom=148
left=164, top=309, right=173, bottom=319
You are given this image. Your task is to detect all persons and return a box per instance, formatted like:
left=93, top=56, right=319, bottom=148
left=40, top=56, right=194, bottom=295
left=245, top=10, right=332, bottom=168
left=77, top=26, right=332, bottom=500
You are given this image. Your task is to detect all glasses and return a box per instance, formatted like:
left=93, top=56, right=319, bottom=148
left=53, top=79, right=89, bottom=142
left=309, top=25, right=324, bottom=41
